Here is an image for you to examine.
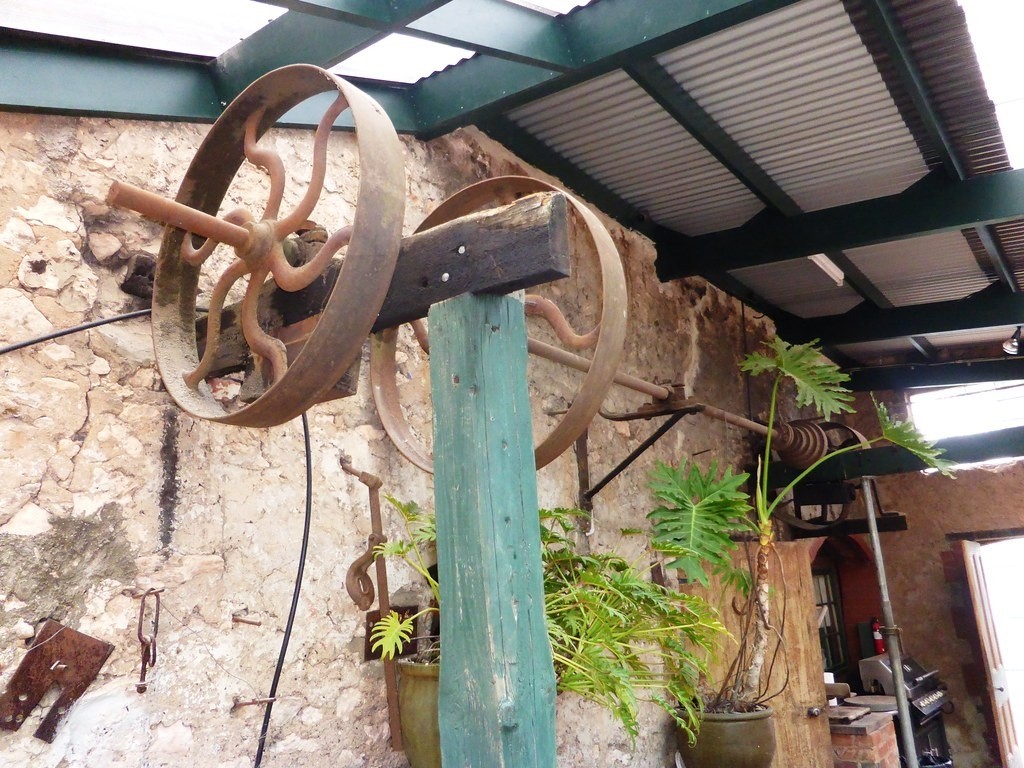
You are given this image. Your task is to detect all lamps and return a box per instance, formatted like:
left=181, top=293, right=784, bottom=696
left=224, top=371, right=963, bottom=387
left=805, top=254, right=845, bottom=286
left=1002, top=327, right=1022, bottom=356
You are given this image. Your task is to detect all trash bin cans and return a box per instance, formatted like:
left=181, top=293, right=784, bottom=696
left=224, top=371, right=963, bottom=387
left=916, top=755, right=952, bottom=768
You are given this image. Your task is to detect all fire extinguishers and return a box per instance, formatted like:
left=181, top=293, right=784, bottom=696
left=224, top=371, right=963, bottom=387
left=872, top=611, right=885, bottom=654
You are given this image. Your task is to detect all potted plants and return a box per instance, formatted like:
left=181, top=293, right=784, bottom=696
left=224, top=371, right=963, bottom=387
left=371, top=489, right=741, bottom=767
left=645, top=337, right=957, bottom=768
